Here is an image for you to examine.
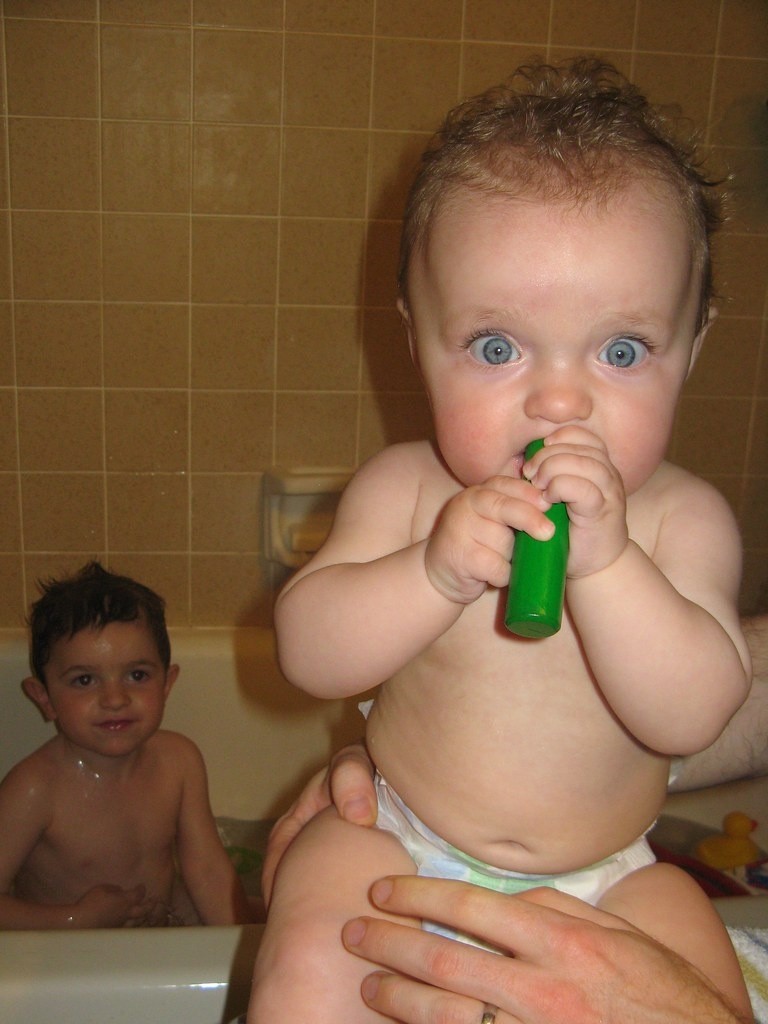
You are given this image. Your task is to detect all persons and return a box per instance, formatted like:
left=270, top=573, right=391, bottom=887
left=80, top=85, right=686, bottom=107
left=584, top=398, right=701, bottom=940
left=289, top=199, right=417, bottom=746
left=0, top=562, right=239, bottom=929
left=241, top=51, right=768, bottom=1024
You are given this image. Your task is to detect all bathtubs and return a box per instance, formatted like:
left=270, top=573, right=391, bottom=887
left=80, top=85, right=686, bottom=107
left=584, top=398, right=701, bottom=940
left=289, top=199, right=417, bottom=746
left=1, top=623, right=768, bottom=1024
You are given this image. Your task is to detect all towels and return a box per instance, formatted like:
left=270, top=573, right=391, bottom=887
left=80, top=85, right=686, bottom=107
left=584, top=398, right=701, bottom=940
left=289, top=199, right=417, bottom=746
left=722, top=922, right=767, bottom=1024
